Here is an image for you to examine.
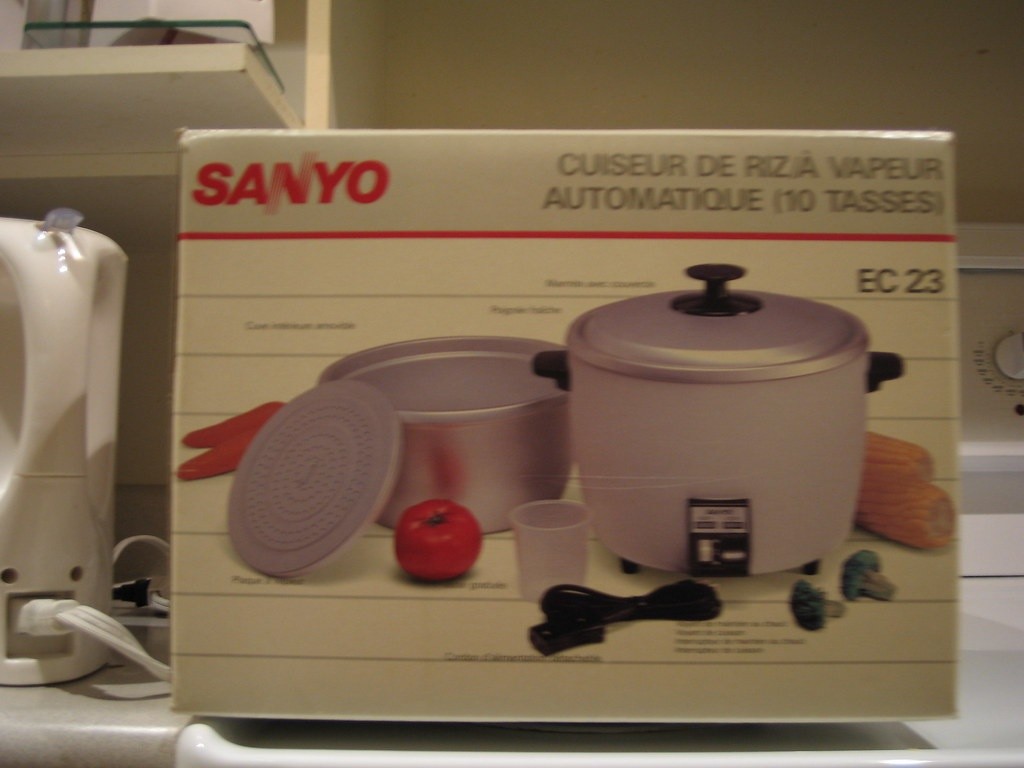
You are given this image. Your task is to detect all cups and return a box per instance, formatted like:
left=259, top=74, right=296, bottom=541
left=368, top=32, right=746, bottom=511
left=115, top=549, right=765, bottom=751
left=512, top=498, right=592, bottom=602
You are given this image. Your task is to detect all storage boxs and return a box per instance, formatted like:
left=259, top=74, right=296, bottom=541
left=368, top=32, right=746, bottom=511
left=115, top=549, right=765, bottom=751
left=170, top=129, right=964, bottom=724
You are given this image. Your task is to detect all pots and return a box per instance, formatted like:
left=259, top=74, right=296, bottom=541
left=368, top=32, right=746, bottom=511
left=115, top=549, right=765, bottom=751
left=532, top=264, right=905, bottom=575
left=319, top=336, right=575, bottom=534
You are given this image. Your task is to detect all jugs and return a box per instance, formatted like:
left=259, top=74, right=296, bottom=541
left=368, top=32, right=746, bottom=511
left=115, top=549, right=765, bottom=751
left=0, top=209, right=129, bottom=685
